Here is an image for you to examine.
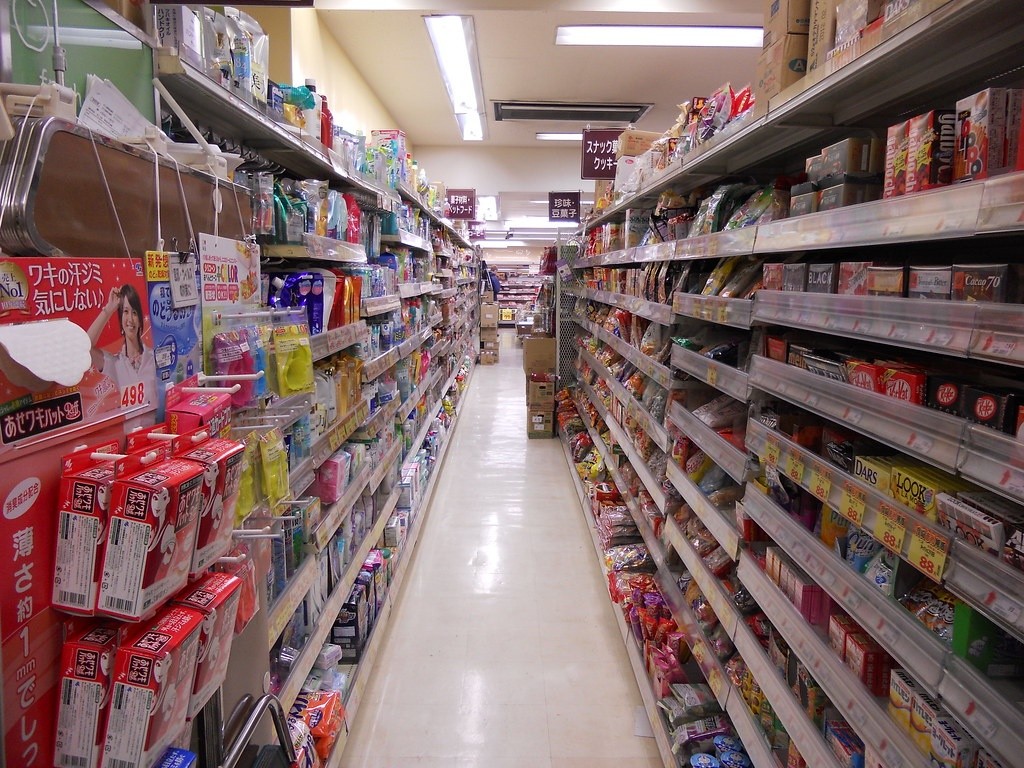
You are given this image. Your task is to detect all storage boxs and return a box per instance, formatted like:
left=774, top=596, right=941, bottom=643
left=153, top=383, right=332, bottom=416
left=523, top=336, right=557, bottom=439
left=480, top=291, right=500, bottom=365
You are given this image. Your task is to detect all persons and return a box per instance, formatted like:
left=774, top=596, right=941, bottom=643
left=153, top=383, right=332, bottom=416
left=489, top=265, right=500, bottom=301
left=86, top=285, right=159, bottom=413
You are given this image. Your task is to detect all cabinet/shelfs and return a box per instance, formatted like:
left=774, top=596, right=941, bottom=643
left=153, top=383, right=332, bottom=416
left=497, top=269, right=545, bottom=324
left=0, top=0, right=480, bottom=768
left=554, top=0, right=1024, bottom=768
